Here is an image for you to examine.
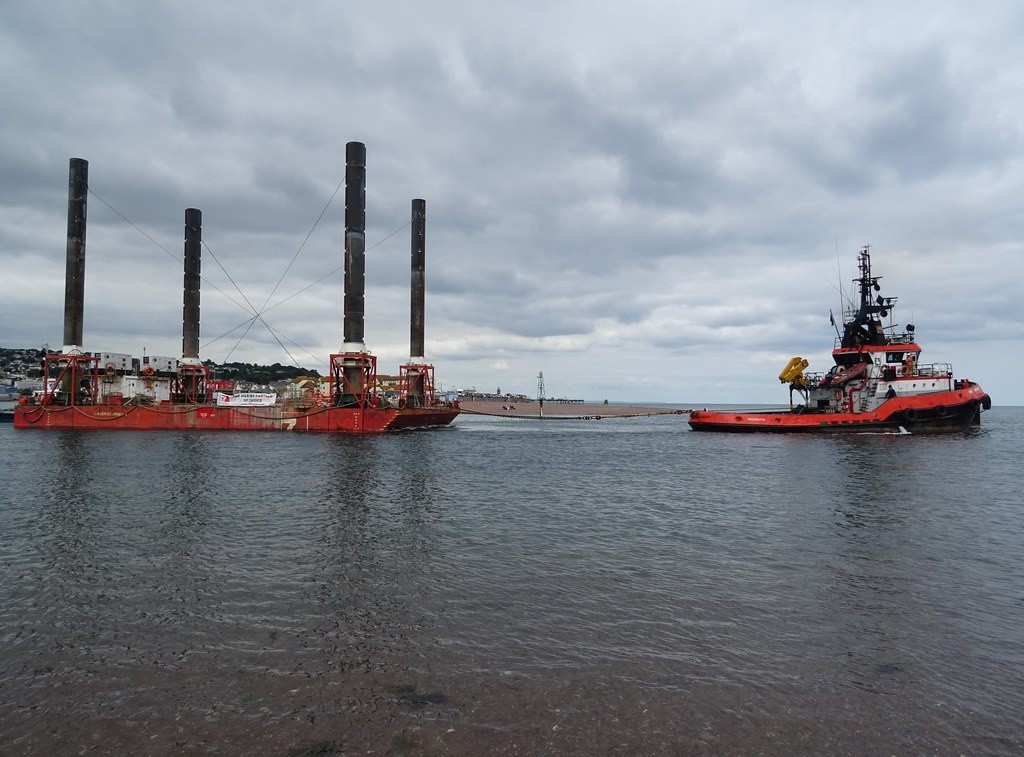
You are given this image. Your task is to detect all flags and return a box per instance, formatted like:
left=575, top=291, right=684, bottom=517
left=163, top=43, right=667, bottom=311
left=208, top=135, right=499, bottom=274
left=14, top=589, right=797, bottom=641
left=830, top=313, right=835, bottom=327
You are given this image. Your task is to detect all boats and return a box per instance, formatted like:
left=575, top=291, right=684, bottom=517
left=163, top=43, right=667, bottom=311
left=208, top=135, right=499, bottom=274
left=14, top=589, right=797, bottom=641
left=687, top=246, right=992, bottom=435
left=14, top=141, right=465, bottom=432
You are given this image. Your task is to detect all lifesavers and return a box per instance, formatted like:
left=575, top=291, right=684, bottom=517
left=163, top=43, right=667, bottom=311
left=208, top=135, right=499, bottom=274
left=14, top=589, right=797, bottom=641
left=900, top=366, right=908, bottom=375
left=834, top=390, right=841, bottom=401
left=144, top=366, right=154, bottom=376
left=880, top=363, right=888, bottom=374
left=313, top=392, right=325, bottom=403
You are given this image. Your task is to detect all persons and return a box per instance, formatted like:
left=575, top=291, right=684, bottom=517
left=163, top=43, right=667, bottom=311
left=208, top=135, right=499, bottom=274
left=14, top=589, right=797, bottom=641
left=906, top=354, right=913, bottom=377
left=885, top=385, right=897, bottom=400
left=964, top=379, right=970, bottom=389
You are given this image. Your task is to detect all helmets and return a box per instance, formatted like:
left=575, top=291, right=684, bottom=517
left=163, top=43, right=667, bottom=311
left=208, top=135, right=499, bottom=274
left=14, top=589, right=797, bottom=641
left=907, top=353, right=911, bottom=355
